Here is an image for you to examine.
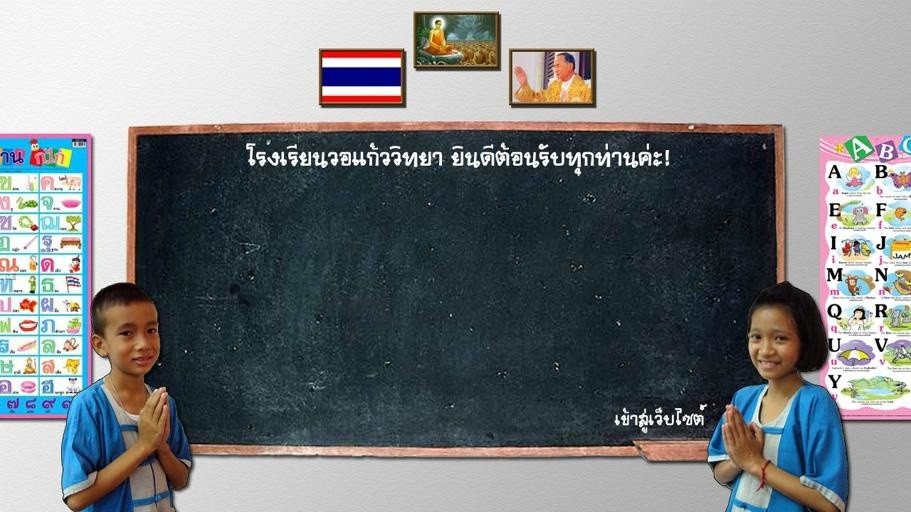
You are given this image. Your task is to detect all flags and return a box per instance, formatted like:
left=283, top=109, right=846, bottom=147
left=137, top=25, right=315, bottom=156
left=66, top=276, right=81, bottom=287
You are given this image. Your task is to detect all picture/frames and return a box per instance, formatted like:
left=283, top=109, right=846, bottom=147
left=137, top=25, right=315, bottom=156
left=507, top=47, right=597, bottom=109
left=317, top=46, right=407, bottom=108
left=412, top=10, right=502, bottom=71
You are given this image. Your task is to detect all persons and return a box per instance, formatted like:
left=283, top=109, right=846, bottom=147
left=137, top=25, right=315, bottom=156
left=514, top=52, right=591, bottom=103
left=69, top=258, right=81, bottom=273
left=706, top=280, right=850, bottom=512
left=426, top=20, right=453, bottom=55
left=846, top=308, right=866, bottom=332
left=29, top=255, right=37, bottom=270
left=846, top=168, right=863, bottom=187
left=60, top=281, right=194, bottom=512
left=28, top=276, right=36, bottom=293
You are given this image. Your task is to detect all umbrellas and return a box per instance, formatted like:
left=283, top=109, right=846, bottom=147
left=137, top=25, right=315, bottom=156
left=839, top=346, right=871, bottom=365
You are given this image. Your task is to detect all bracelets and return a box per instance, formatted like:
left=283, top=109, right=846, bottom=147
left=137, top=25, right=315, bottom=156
left=755, top=459, right=772, bottom=492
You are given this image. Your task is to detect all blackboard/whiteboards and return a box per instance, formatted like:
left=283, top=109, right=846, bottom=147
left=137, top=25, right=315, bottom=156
left=132, top=123, right=784, bottom=448
